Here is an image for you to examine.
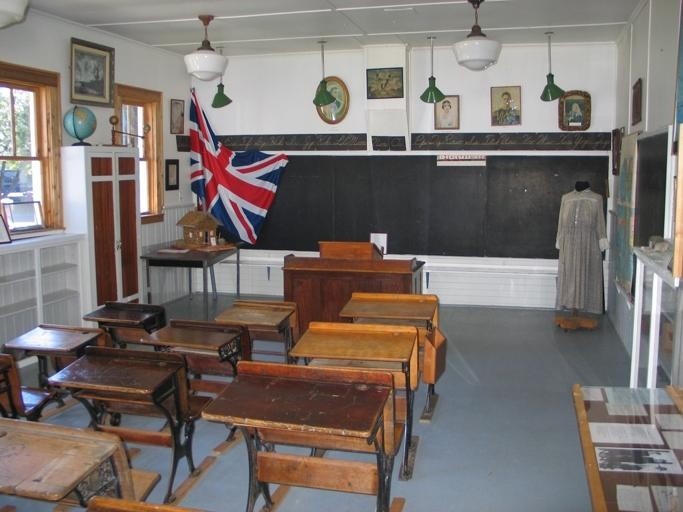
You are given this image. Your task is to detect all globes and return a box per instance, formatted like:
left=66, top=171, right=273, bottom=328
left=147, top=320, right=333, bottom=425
left=63, top=105, right=97, bottom=146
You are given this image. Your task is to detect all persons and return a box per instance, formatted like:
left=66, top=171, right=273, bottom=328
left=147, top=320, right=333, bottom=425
left=439, top=101, right=455, bottom=129
left=567, top=104, right=583, bottom=126
left=329, top=87, right=343, bottom=107
left=554, top=182, right=607, bottom=318
left=492, top=94, right=518, bottom=123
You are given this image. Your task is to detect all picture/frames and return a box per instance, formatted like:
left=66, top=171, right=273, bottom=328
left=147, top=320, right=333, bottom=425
left=71, top=37, right=115, bottom=108
left=165, top=159, right=179, bottom=190
left=491, top=86, right=521, bottom=126
left=558, top=90, right=591, bottom=131
left=315, top=76, right=350, bottom=124
left=611, top=129, right=621, bottom=177
left=171, top=98, right=184, bottom=135
left=366, top=67, right=403, bottom=99
left=434, top=95, right=459, bottom=130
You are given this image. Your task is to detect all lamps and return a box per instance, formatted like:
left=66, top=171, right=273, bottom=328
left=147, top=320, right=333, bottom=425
left=452, top=0, right=502, bottom=71
left=212, top=48, right=232, bottom=108
left=313, top=41, right=336, bottom=106
left=420, top=37, right=445, bottom=103
left=183, top=15, right=228, bottom=82
left=540, top=32, right=565, bottom=101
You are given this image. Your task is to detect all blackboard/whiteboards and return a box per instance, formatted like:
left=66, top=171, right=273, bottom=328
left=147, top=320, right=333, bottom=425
left=215, top=155, right=608, bottom=260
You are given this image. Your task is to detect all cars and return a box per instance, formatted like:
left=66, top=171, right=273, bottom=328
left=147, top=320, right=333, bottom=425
left=0, top=169, right=32, bottom=201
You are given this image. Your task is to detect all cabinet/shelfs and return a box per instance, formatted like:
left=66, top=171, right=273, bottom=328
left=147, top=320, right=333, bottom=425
left=1, top=229, right=85, bottom=350
left=60, top=145, right=144, bottom=327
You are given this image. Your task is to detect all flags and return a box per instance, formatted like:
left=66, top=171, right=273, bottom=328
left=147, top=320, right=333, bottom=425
left=189, top=88, right=290, bottom=248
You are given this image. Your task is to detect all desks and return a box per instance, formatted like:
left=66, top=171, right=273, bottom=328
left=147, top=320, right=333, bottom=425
left=5, top=320, right=93, bottom=379
left=572, top=383, right=682, bottom=512
left=161, top=319, right=246, bottom=378
left=140, top=241, right=245, bottom=321
left=222, top=357, right=403, bottom=512
left=340, top=292, right=443, bottom=408
left=300, top=319, right=423, bottom=478
left=71, top=348, right=205, bottom=475
left=91, top=296, right=161, bottom=345
left=227, top=299, right=294, bottom=353
left=3, top=415, right=155, bottom=512
